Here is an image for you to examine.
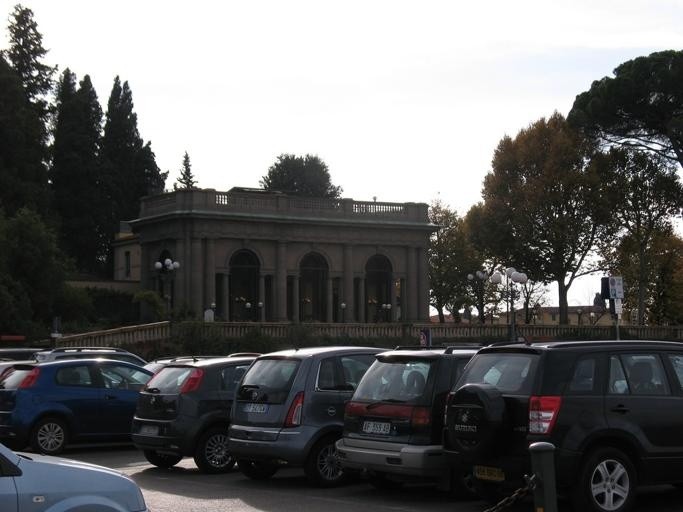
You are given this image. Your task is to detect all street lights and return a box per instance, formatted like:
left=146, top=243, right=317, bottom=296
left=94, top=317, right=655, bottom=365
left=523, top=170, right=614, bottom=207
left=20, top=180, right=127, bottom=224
left=366, top=297, right=378, bottom=321
left=299, top=296, right=310, bottom=320
left=234, top=296, right=246, bottom=321
left=339, top=302, right=347, bottom=322
left=153, top=258, right=179, bottom=321
left=243, top=298, right=265, bottom=322
left=210, top=301, right=216, bottom=322
left=490, top=265, right=528, bottom=334
left=382, top=302, right=394, bottom=321
left=531, top=305, right=537, bottom=324
left=466, top=269, right=490, bottom=328
left=576, top=308, right=582, bottom=325
left=590, top=311, right=595, bottom=325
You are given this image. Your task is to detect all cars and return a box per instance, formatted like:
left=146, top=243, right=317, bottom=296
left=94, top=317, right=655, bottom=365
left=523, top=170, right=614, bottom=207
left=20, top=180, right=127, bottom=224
left=0, top=439, right=146, bottom=512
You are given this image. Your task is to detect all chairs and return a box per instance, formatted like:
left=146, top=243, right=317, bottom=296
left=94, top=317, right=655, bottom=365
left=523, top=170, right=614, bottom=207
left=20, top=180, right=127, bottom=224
left=625, top=362, right=657, bottom=394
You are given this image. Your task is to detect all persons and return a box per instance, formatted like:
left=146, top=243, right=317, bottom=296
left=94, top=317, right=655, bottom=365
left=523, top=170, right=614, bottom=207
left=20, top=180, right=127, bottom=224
left=203, top=302, right=217, bottom=321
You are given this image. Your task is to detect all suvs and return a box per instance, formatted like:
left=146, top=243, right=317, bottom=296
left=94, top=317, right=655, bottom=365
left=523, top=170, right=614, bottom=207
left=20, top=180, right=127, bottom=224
left=130, top=350, right=266, bottom=475
left=224, top=345, right=397, bottom=490
left=332, top=340, right=499, bottom=501
left=1, top=345, right=179, bottom=455
left=442, top=336, right=683, bottom=512
